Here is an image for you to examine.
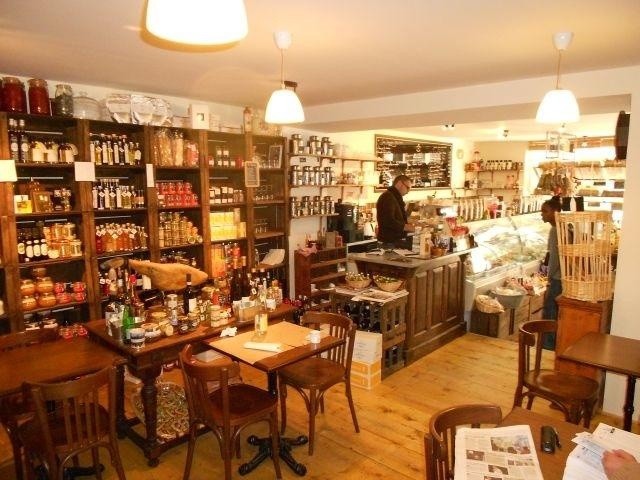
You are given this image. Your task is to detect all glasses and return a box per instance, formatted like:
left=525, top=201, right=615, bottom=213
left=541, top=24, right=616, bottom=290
left=534, top=30, right=581, bottom=127
left=263, top=30, right=306, bottom=126
left=139, top=2, right=250, bottom=53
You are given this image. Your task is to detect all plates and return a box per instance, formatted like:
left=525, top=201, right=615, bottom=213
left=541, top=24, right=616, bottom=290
left=462, top=212, right=550, bottom=333
left=295, top=247, right=348, bottom=307
left=82, top=117, right=209, bottom=322
left=0, top=109, right=98, bottom=354
left=202, top=128, right=293, bottom=301
left=349, top=249, right=476, bottom=369
left=288, top=151, right=379, bottom=216
left=476, top=169, right=523, bottom=192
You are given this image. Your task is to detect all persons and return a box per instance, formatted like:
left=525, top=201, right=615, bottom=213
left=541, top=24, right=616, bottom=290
left=540, top=201, right=573, bottom=350
left=601, top=447, right=636, bottom=477
left=376, top=174, right=426, bottom=247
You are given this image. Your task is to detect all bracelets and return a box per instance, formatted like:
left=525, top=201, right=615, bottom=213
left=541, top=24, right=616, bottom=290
left=374, top=276, right=403, bottom=291
left=490, top=287, right=529, bottom=308
left=346, top=276, right=372, bottom=288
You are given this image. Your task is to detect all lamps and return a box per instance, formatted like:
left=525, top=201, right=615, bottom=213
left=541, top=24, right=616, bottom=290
left=422, top=403, right=503, bottom=479
left=277, top=310, right=361, bottom=454
left=178, top=343, right=282, bottom=476
left=0, top=326, right=63, bottom=478
left=513, top=319, right=602, bottom=430
left=15, top=362, right=127, bottom=480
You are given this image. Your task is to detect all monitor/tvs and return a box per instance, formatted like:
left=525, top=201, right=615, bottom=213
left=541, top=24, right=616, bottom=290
left=563, top=421, right=639, bottom=480
left=455, top=424, right=542, bottom=480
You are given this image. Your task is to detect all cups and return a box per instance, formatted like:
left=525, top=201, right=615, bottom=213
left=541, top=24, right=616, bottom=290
left=401, top=181, right=411, bottom=191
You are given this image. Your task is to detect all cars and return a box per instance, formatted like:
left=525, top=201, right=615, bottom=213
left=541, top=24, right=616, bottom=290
left=305, top=329, right=322, bottom=344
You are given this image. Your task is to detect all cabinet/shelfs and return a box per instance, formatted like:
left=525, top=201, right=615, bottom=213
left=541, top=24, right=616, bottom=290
left=176, top=312, right=198, bottom=335
left=209, top=186, right=244, bottom=204
left=246, top=267, right=284, bottom=300
left=105, top=285, right=146, bottom=350
left=292, top=134, right=304, bottom=155
left=335, top=236, right=342, bottom=247
left=289, top=195, right=335, bottom=217
left=158, top=212, right=202, bottom=247
left=221, top=242, right=250, bottom=301
left=19, top=265, right=87, bottom=311
left=98, top=263, right=143, bottom=296
left=89, top=133, right=142, bottom=166
left=309, top=136, right=323, bottom=155
left=210, top=208, right=246, bottom=241
left=27, top=78, right=51, bottom=118
left=165, top=294, right=178, bottom=328
left=346, top=272, right=370, bottom=282
left=17, top=219, right=82, bottom=264
left=243, top=106, right=253, bottom=133
left=291, top=165, right=336, bottom=186
left=504, top=175, right=514, bottom=190
left=183, top=273, right=197, bottom=317
left=254, top=285, right=276, bottom=338
left=321, top=137, right=334, bottom=157
left=291, top=295, right=310, bottom=328
left=7, top=118, right=74, bottom=165
left=486, top=160, right=517, bottom=171
left=95, top=222, right=148, bottom=256
left=91, top=178, right=137, bottom=210
left=522, top=195, right=543, bottom=214
left=506, top=199, right=521, bottom=217
left=457, top=197, right=483, bottom=221
left=52, top=84, right=74, bottom=119
left=141, top=257, right=152, bottom=291
left=208, top=145, right=245, bottom=169
left=154, top=181, right=199, bottom=207
left=2, top=76, right=27, bottom=116
left=24, top=318, right=58, bottom=345
left=252, top=185, right=280, bottom=201
left=159, top=250, right=197, bottom=269
left=335, top=298, right=400, bottom=334
